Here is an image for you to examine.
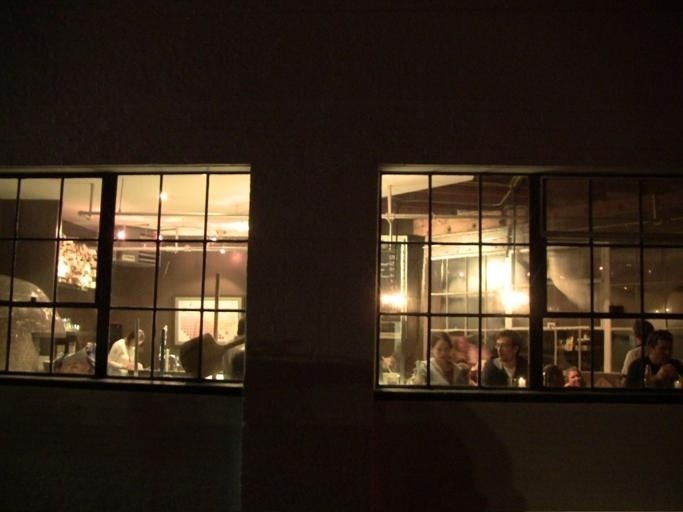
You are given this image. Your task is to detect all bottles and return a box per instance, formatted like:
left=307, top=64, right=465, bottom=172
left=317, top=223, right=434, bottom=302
left=641, top=365, right=652, bottom=388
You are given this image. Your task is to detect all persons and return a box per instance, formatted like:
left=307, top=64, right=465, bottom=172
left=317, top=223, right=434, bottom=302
left=409, top=320, right=683, bottom=389
left=105, top=329, right=145, bottom=378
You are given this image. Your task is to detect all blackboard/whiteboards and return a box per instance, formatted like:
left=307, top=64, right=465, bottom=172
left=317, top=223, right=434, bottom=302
left=379, top=234, right=408, bottom=315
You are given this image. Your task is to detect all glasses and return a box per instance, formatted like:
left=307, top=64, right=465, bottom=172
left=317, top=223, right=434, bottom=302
left=493, top=342, right=513, bottom=348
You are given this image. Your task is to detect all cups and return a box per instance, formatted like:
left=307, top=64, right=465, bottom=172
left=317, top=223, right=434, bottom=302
left=382, top=373, right=397, bottom=387
left=674, top=379, right=682, bottom=389
